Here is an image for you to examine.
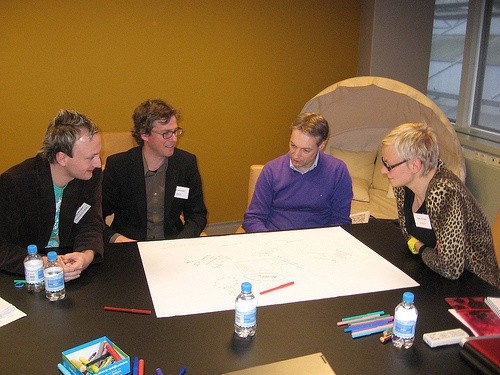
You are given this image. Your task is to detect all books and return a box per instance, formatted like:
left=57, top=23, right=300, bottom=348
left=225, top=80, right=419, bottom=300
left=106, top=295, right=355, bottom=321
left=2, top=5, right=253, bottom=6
left=447, top=292, right=500, bottom=375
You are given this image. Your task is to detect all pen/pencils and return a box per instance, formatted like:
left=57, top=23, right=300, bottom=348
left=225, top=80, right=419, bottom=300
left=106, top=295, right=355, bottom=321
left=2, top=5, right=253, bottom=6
left=103, top=306, right=152, bottom=314
left=337, top=311, right=393, bottom=342
left=180, top=368, right=187, bottom=375
left=133, top=357, right=137, bottom=375
left=57, top=363, right=72, bottom=375
left=261, top=281, right=295, bottom=294
left=155, top=368, right=163, bottom=375
left=139, top=359, right=144, bottom=375
left=14, top=279, right=27, bottom=284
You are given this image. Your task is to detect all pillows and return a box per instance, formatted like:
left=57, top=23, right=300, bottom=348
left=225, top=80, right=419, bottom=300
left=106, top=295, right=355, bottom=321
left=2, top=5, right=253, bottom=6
left=373, top=146, right=393, bottom=192
left=329, top=145, right=378, bottom=203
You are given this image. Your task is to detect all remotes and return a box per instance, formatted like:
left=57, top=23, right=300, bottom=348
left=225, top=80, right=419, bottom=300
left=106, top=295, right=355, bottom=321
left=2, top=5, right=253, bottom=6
left=422, top=328, right=470, bottom=348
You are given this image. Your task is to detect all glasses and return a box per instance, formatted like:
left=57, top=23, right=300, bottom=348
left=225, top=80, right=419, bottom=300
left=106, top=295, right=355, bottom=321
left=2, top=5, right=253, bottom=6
left=151, top=127, right=183, bottom=139
left=381, top=157, right=408, bottom=171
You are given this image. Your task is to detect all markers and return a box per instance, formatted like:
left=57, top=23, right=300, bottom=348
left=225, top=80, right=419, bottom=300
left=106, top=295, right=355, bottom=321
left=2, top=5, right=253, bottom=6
left=71, top=343, right=122, bottom=375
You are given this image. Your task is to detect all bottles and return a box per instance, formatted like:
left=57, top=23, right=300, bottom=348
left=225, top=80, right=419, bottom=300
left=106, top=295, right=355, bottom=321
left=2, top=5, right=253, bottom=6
left=24, top=245, right=45, bottom=293
left=43, top=251, right=66, bottom=301
left=233, top=282, right=258, bottom=338
left=392, top=292, right=418, bottom=349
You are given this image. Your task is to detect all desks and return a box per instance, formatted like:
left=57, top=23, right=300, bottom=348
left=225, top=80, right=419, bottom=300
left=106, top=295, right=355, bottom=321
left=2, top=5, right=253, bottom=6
left=0, top=217, right=500, bottom=375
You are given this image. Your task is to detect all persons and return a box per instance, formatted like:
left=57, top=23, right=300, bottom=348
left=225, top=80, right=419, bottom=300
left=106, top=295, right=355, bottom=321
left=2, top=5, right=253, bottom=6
left=99, top=95, right=210, bottom=244
left=1, top=109, right=105, bottom=284
left=379, top=122, right=500, bottom=290
left=241, top=110, right=353, bottom=232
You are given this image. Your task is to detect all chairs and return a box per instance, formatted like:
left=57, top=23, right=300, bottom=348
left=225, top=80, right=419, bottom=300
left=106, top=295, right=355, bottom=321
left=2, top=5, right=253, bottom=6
left=235, top=163, right=266, bottom=233
